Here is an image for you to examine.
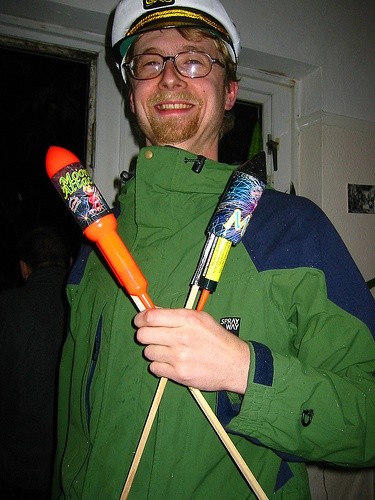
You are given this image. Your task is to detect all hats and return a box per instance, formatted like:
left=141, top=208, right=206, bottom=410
left=111, top=0, right=241, bottom=84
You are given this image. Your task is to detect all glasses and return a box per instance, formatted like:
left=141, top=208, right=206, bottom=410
left=123, top=51, right=230, bottom=81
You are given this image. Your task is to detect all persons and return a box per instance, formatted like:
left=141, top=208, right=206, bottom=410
left=53, top=0, right=374, bottom=500
left=0, top=225, right=74, bottom=500
